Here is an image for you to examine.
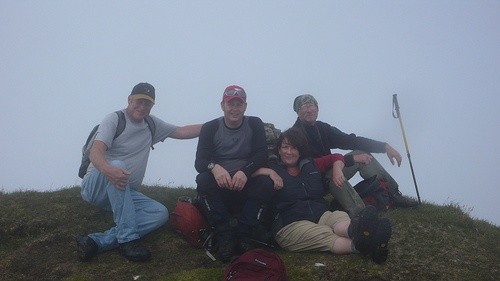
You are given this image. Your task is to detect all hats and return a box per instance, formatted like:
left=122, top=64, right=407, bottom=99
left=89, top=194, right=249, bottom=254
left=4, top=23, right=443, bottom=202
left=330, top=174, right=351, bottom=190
left=293, top=94, right=318, bottom=113
left=223, top=85, right=246, bottom=103
left=131, top=83, right=155, bottom=104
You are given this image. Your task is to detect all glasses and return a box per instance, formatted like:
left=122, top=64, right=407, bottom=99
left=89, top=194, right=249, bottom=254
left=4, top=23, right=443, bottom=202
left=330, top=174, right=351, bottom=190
left=223, top=90, right=246, bottom=98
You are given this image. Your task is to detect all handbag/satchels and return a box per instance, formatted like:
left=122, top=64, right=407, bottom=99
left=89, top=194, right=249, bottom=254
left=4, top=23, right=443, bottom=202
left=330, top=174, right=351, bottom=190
left=248, top=115, right=281, bottom=163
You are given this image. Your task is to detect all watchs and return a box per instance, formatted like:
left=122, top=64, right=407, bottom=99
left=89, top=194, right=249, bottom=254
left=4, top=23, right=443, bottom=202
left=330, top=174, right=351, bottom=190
left=207, top=162, right=216, bottom=171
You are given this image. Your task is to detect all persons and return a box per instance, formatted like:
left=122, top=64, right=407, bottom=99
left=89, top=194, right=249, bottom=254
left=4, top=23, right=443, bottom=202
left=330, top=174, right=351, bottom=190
left=195, top=85, right=417, bottom=263
left=77, top=83, right=206, bottom=262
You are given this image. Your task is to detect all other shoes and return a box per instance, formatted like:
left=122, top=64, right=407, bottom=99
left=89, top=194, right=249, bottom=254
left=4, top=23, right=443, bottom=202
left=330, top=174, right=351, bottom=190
left=74, top=235, right=99, bottom=262
left=350, top=205, right=374, bottom=235
left=386, top=190, right=412, bottom=207
left=119, top=241, right=152, bottom=262
left=368, top=218, right=392, bottom=263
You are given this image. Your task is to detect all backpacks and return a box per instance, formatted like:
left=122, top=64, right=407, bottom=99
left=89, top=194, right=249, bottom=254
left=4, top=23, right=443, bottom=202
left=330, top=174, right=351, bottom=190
left=221, top=248, right=289, bottom=281
left=79, top=111, right=155, bottom=179
left=332, top=174, right=395, bottom=212
